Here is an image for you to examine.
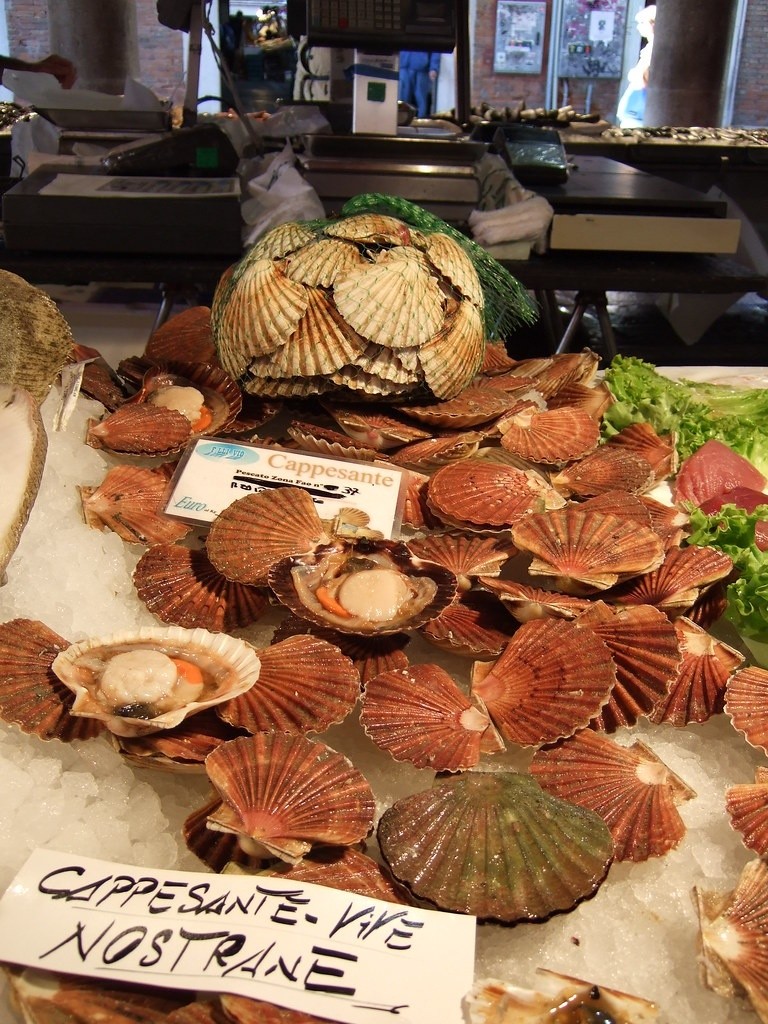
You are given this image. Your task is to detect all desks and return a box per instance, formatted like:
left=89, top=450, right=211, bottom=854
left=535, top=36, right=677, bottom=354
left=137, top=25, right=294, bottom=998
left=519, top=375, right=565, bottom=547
left=0, top=254, right=768, bottom=370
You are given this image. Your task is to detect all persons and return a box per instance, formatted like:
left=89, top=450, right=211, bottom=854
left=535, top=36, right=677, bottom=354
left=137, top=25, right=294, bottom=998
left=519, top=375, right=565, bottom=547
left=398, top=50, right=440, bottom=118
left=0, top=53, right=80, bottom=90
left=616, top=5, right=657, bottom=128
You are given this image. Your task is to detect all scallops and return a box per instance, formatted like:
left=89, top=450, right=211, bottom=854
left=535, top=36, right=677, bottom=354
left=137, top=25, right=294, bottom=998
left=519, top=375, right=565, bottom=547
left=1, top=208, right=768, bottom=1021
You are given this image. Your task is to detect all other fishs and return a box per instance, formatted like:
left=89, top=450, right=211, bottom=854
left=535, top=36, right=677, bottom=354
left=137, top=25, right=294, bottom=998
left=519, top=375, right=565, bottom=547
left=437, top=108, right=766, bottom=146
